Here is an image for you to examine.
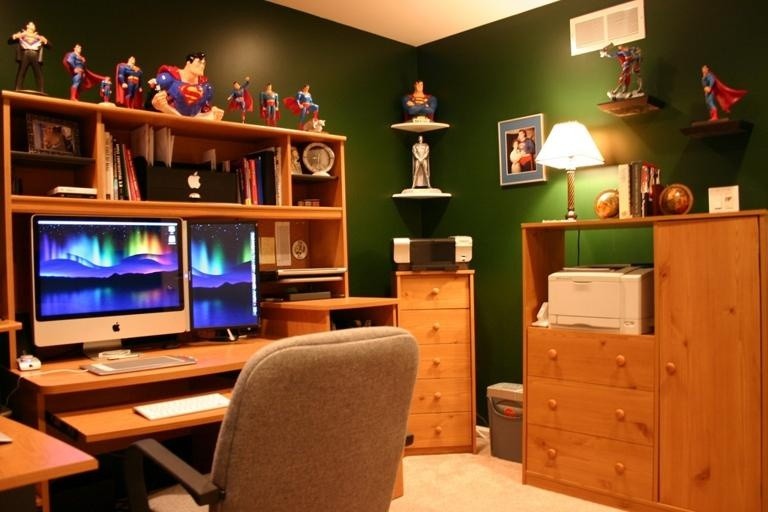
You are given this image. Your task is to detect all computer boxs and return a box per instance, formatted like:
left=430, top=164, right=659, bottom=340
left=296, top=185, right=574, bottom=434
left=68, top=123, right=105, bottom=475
left=145, top=166, right=239, bottom=202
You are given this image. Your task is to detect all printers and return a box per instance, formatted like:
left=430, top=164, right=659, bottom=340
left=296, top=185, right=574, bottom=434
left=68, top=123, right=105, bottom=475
left=547, top=264, right=653, bottom=336
left=391, top=236, right=473, bottom=272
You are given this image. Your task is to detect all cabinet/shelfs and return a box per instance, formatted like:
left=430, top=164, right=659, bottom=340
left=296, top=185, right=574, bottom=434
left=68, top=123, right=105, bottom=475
left=395, top=269, right=477, bottom=457
left=521, top=218, right=656, bottom=512
left=260, top=296, right=404, bottom=501
left=0, top=90, right=350, bottom=371
left=390, top=123, right=452, bottom=199
left=654, top=209, right=768, bottom=512
left=597, top=94, right=754, bottom=140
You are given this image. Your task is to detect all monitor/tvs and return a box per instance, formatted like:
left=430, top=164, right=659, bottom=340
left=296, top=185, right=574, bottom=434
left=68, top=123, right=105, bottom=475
left=187, top=221, right=260, bottom=341
left=30, top=213, right=190, bottom=362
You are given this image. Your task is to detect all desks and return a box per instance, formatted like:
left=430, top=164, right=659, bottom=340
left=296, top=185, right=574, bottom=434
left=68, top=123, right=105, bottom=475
left=0, top=413, right=99, bottom=512
left=13, top=335, right=285, bottom=512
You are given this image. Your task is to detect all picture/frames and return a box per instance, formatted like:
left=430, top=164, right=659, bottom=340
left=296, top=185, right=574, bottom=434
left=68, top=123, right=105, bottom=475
left=497, top=113, right=547, bottom=187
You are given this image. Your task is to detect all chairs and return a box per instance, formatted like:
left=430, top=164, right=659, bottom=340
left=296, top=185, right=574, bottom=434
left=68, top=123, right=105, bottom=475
left=126, top=326, right=419, bottom=512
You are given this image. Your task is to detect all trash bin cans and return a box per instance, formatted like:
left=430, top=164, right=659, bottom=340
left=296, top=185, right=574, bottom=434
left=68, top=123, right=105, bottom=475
left=484, top=382, right=523, bottom=463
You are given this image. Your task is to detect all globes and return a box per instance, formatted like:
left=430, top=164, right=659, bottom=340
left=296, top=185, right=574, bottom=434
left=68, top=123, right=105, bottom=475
left=659, top=183, right=694, bottom=215
left=593, top=188, right=619, bottom=218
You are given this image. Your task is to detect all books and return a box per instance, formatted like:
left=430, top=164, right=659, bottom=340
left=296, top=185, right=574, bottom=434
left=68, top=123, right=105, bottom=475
left=107, top=123, right=285, bottom=203
left=616, top=159, right=662, bottom=220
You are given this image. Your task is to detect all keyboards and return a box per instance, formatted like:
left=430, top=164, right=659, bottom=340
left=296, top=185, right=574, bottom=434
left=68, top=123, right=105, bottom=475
left=80, top=355, right=197, bottom=376
left=133, top=392, right=230, bottom=422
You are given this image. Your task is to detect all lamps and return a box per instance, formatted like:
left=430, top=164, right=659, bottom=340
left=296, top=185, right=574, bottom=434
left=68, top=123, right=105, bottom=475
left=535, top=121, right=605, bottom=221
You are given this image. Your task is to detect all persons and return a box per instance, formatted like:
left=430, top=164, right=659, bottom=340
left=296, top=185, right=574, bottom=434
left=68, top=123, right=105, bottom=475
left=411, top=135, right=432, bottom=188
left=63, top=42, right=105, bottom=100
left=98, top=75, right=112, bottom=100
left=402, top=80, right=437, bottom=123
left=11, top=22, right=54, bottom=96
left=260, top=82, right=279, bottom=124
left=284, top=83, right=325, bottom=131
left=148, top=49, right=223, bottom=119
left=117, top=56, right=144, bottom=108
left=505, top=126, right=536, bottom=174
left=227, top=77, right=251, bottom=122
left=701, top=65, right=749, bottom=122
left=601, top=43, right=647, bottom=100
